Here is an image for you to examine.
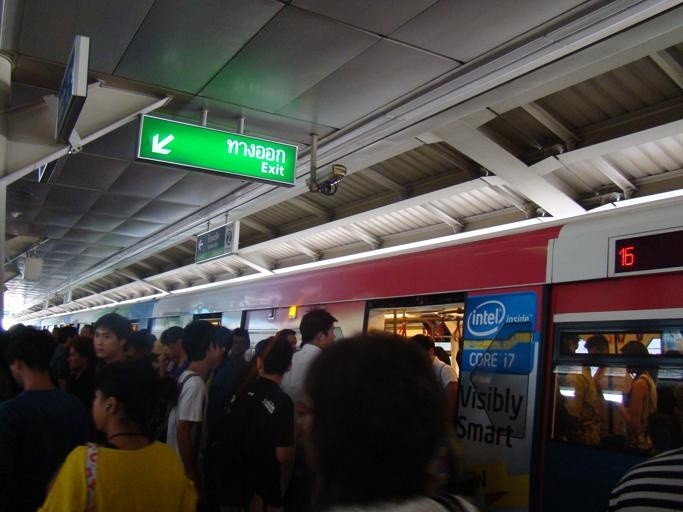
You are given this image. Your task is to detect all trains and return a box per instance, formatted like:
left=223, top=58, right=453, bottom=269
left=1, top=185, right=683, bottom=512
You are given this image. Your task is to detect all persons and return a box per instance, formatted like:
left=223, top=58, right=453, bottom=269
left=553, top=333, right=682, bottom=510
left=0, top=307, right=479, bottom=511
